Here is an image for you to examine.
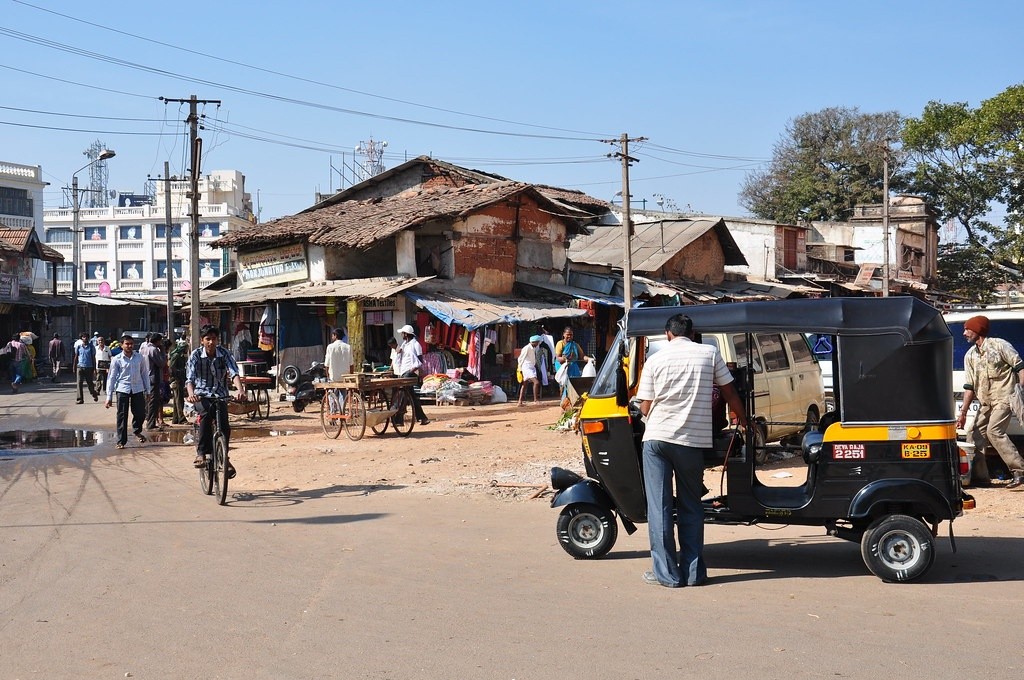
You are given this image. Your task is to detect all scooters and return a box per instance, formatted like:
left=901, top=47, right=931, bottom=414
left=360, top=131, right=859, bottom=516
left=282, top=363, right=328, bottom=412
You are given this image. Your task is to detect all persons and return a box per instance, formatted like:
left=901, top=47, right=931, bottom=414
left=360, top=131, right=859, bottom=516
left=517, top=334, right=543, bottom=408
left=956, top=315, right=1024, bottom=488
left=72, top=331, right=194, bottom=449
left=185, top=325, right=246, bottom=479
left=6, top=333, right=31, bottom=394
left=636, top=314, right=756, bottom=587
left=93, top=224, right=213, bottom=281
left=387, top=324, right=430, bottom=427
left=325, top=328, right=354, bottom=426
left=49, top=332, right=65, bottom=383
left=554, top=326, right=596, bottom=400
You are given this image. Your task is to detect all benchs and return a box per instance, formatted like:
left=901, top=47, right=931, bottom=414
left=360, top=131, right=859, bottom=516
left=801, top=412, right=842, bottom=462
left=705, top=365, right=748, bottom=460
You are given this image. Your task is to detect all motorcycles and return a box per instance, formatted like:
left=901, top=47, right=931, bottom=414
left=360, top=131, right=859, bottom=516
left=548, top=296, right=977, bottom=583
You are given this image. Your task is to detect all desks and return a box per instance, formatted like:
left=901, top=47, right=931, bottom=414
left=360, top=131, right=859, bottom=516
left=236, top=361, right=269, bottom=391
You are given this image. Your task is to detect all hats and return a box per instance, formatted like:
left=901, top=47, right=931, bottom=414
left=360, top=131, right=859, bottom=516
left=529, top=335, right=542, bottom=342
left=397, top=325, right=416, bottom=336
left=964, top=316, right=989, bottom=336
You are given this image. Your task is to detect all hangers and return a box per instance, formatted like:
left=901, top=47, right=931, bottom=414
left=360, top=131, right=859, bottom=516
left=540, top=326, right=550, bottom=336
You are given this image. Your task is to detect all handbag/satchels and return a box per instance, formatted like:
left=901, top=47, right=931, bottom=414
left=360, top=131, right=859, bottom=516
left=517, top=369, right=522, bottom=383
left=554, top=362, right=567, bottom=385
left=581, top=359, right=596, bottom=378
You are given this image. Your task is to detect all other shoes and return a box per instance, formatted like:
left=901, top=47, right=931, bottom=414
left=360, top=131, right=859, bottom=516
left=76, top=400, right=84, bottom=404
left=52, top=377, right=56, bottom=382
left=11, top=383, right=19, bottom=393
left=159, top=422, right=171, bottom=427
left=92, top=394, right=99, bottom=402
left=643, top=570, right=679, bottom=587
left=180, top=421, right=193, bottom=426
left=420, top=420, right=430, bottom=425
left=145, top=427, right=161, bottom=432
left=391, top=424, right=403, bottom=427
left=1006, top=475, right=1024, bottom=488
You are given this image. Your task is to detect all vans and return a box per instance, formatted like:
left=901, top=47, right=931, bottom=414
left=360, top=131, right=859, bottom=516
left=941, top=309, right=1024, bottom=439
left=626, top=332, right=826, bottom=467
left=804, top=331, right=836, bottom=414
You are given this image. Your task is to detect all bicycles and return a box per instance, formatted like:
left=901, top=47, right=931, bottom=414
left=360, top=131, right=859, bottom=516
left=196, top=396, right=235, bottom=506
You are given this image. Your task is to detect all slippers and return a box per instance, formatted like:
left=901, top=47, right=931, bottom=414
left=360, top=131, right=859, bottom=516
left=193, top=456, right=206, bottom=466
left=225, top=461, right=236, bottom=479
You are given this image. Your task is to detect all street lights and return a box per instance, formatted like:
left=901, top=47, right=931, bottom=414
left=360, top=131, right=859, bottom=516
left=71, top=150, right=116, bottom=365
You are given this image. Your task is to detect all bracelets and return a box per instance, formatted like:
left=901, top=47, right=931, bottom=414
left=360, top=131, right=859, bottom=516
left=961, top=410, right=967, bottom=413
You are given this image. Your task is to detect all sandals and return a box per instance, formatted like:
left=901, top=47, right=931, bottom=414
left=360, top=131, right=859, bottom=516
left=136, top=434, right=146, bottom=444
left=116, top=444, right=124, bottom=449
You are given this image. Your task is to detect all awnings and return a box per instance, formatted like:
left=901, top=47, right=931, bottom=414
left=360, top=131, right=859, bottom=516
left=6, top=298, right=183, bottom=307
left=401, top=287, right=674, bottom=331
left=185, top=276, right=435, bottom=304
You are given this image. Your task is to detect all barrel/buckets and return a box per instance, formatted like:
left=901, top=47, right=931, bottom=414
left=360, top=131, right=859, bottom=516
left=955, top=427, right=976, bottom=486
